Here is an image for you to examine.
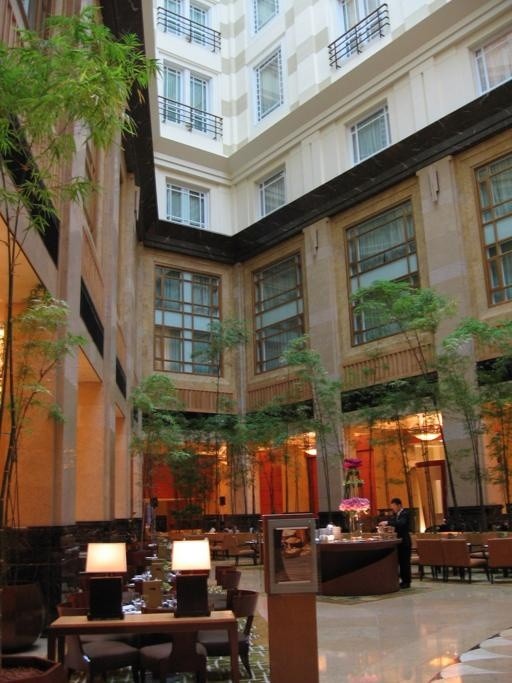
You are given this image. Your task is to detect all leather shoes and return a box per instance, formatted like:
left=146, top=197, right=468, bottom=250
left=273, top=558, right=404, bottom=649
left=401, top=583, right=410, bottom=588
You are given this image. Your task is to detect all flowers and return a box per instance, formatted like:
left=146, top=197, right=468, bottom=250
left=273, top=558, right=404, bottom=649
left=338, top=497, right=373, bottom=515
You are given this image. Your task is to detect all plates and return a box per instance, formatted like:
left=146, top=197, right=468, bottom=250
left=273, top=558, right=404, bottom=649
left=315, top=526, right=341, bottom=542
left=334, top=538, right=380, bottom=544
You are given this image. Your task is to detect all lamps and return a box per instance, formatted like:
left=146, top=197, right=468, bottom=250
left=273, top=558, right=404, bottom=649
left=83, top=537, right=211, bottom=578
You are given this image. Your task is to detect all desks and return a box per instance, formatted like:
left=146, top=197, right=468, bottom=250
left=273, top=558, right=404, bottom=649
left=51, top=610, right=240, bottom=683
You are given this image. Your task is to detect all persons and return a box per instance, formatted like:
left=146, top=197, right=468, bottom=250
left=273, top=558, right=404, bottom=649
left=377, top=496, right=413, bottom=589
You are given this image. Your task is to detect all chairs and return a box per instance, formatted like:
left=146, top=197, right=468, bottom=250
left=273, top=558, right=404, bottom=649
left=212, top=534, right=258, bottom=566
left=58, top=566, right=264, bottom=682
left=408, top=531, right=512, bottom=585
